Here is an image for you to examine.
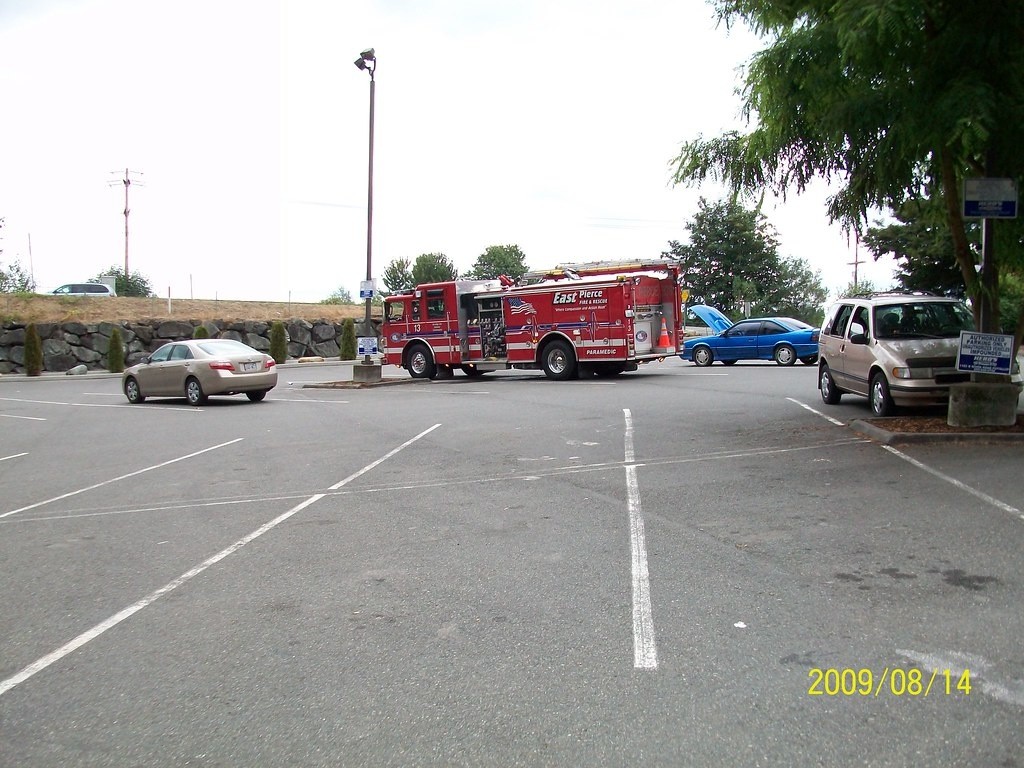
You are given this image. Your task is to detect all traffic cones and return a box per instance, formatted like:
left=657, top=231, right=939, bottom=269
left=657, top=315, right=673, bottom=348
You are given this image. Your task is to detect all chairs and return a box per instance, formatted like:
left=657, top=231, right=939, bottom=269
left=840, top=315, right=868, bottom=337
left=882, top=313, right=900, bottom=333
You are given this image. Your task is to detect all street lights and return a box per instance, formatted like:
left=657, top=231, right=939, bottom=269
left=353, top=46, right=385, bottom=383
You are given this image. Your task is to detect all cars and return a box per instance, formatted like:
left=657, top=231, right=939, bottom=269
left=121, top=338, right=280, bottom=408
left=678, top=305, right=821, bottom=367
left=45, top=282, right=117, bottom=298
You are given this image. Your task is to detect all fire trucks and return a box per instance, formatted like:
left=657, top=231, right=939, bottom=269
left=377, top=259, right=688, bottom=382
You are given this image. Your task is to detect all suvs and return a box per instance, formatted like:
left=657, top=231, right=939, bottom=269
left=816, top=291, right=983, bottom=419
